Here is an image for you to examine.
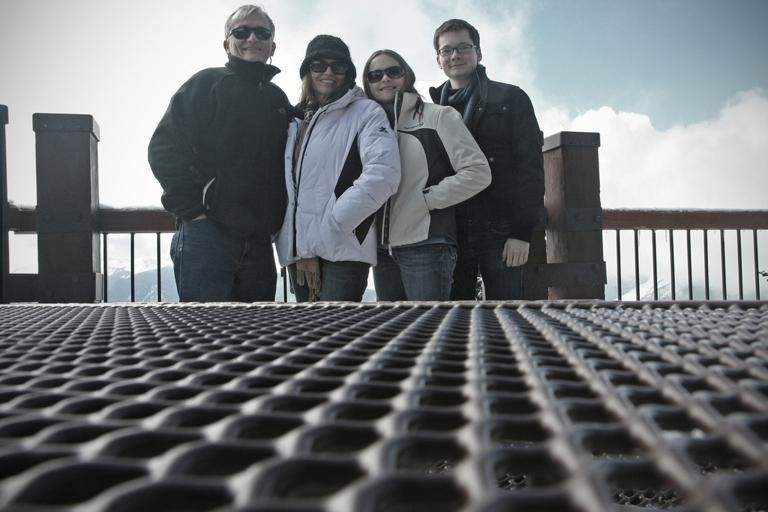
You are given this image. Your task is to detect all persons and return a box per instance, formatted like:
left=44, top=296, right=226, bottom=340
left=363, top=49, right=491, bottom=302
left=273, top=33, right=401, bottom=302
left=147, top=3, right=293, bottom=303
left=430, top=18, right=549, bottom=299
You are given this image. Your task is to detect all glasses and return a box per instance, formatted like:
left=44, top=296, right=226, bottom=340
left=437, top=45, right=480, bottom=53
left=366, top=66, right=405, bottom=83
left=228, top=28, right=271, bottom=41
left=308, top=61, right=351, bottom=75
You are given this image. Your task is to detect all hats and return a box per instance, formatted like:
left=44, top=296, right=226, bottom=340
left=300, top=35, right=357, bottom=80
